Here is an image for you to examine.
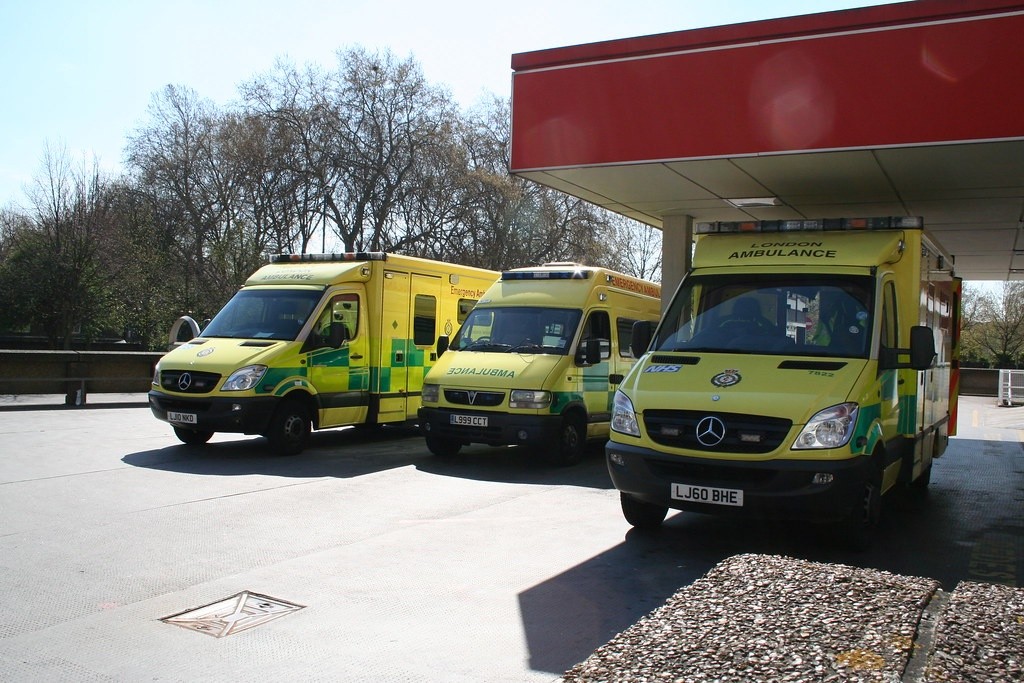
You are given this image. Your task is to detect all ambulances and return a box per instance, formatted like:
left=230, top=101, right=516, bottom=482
left=147, top=249, right=502, bottom=456
left=415, top=262, right=663, bottom=461
left=604, top=218, right=959, bottom=542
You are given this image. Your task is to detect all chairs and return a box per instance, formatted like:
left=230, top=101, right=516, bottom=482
left=713, top=296, right=779, bottom=338
